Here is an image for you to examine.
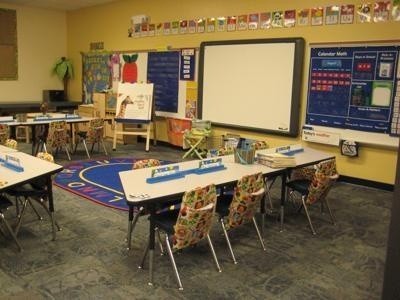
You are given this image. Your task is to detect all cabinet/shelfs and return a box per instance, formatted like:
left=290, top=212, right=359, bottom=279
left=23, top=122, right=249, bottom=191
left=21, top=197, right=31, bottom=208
left=91, top=93, right=109, bottom=138
left=106, top=109, right=139, bottom=146
left=73, top=109, right=93, bottom=133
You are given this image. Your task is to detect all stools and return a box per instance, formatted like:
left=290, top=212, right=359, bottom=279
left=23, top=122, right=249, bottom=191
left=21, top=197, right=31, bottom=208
left=181, top=133, right=205, bottom=159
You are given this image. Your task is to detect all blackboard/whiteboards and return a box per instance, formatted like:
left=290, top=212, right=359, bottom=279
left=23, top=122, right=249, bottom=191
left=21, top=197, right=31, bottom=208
left=80, top=50, right=196, bottom=122
left=309, top=42, right=399, bottom=151
left=196, top=38, right=304, bottom=138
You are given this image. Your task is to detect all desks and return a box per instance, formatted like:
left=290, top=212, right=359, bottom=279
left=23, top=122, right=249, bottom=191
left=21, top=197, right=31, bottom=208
left=1, top=118, right=111, bottom=154
left=118, top=144, right=335, bottom=286
left=7, top=112, right=80, bottom=120
left=0, top=143, right=65, bottom=245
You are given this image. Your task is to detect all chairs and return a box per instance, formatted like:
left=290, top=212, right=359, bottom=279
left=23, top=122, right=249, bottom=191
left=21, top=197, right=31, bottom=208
left=9, top=152, right=62, bottom=236
left=72, top=117, right=110, bottom=160
left=11, top=112, right=31, bottom=143
left=206, top=146, right=236, bottom=158
left=131, top=158, right=169, bottom=257
left=251, top=141, right=268, bottom=151
left=0, top=124, right=13, bottom=143
left=146, top=183, right=222, bottom=292
left=216, top=171, right=267, bottom=265
left=287, top=157, right=338, bottom=235
left=36, top=119, right=72, bottom=163
left=4, top=139, right=18, bottom=149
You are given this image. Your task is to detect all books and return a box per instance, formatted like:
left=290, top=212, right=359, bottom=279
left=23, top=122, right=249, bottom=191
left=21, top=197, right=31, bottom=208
left=256, top=153, right=297, bottom=168
